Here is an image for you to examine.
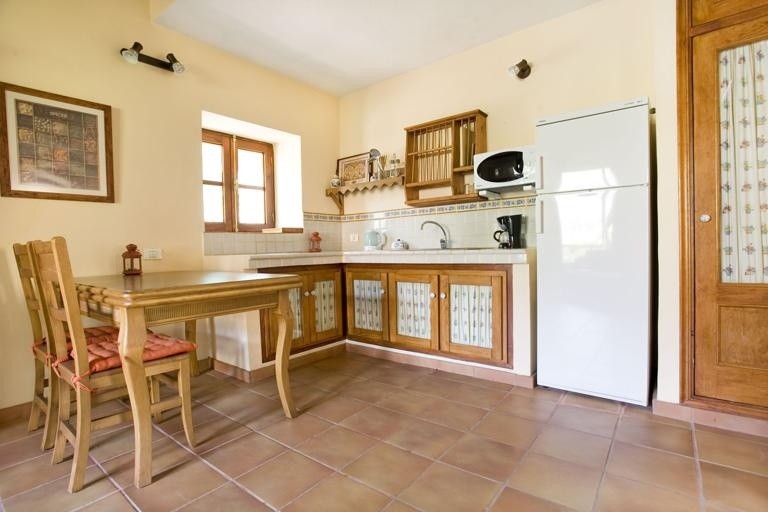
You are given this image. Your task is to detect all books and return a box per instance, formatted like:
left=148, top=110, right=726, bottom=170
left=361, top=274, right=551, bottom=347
left=459, top=127, right=474, bottom=167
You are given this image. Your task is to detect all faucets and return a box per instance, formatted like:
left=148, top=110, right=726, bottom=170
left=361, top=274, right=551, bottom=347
left=420, top=221, right=448, bottom=249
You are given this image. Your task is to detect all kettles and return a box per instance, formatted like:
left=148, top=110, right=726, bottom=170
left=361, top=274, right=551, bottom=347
left=363, top=227, right=386, bottom=251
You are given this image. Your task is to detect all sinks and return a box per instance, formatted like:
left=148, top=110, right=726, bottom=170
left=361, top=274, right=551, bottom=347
left=390, top=248, right=493, bottom=250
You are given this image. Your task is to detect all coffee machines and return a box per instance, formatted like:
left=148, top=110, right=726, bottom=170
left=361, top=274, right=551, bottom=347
left=493, top=214, right=522, bottom=250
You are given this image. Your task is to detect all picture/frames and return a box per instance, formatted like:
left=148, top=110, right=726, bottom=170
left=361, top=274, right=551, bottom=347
left=336, top=152, right=371, bottom=183
left=0, top=80, right=114, bottom=204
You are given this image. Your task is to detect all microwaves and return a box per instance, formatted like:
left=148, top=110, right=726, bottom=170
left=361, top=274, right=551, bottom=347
left=473, top=143, right=537, bottom=193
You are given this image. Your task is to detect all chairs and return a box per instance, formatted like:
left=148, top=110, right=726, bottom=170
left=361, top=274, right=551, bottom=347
left=12, top=233, right=199, bottom=493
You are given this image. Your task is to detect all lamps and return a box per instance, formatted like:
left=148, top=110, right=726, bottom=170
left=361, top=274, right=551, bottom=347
left=120, top=41, right=185, bottom=76
left=508, top=57, right=530, bottom=79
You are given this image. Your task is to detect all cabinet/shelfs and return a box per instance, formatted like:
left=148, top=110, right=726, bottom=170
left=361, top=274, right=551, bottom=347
left=402, top=109, right=488, bottom=207
left=203, top=263, right=342, bottom=383
left=342, top=263, right=390, bottom=360
left=389, top=263, right=535, bottom=393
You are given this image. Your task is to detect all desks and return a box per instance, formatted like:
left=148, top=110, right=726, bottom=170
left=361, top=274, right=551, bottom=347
left=53, top=269, right=304, bottom=490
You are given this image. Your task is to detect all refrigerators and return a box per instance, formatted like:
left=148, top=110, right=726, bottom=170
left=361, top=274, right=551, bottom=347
left=535, top=96, right=657, bottom=407
